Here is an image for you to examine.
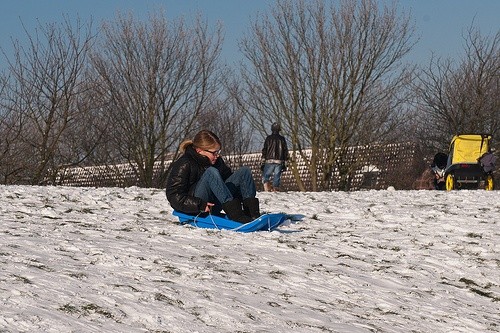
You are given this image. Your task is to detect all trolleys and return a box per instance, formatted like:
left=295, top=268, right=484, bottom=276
left=444, top=134, right=496, bottom=191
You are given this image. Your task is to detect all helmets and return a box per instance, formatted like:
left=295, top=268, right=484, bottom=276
left=271, top=122, right=281, bottom=131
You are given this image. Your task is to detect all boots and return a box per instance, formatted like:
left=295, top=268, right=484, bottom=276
left=264, top=182, right=279, bottom=193
left=222, top=198, right=261, bottom=224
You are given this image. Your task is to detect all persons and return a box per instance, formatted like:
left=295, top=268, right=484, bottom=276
left=166, top=130, right=261, bottom=224
left=261, top=122, right=289, bottom=192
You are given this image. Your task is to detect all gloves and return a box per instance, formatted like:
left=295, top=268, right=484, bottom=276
left=283, top=161, right=288, bottom=171
left=259, top=158, right=265, bottom=171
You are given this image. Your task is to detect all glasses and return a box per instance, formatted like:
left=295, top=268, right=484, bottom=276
left=203, top=149, right=221, bottom=156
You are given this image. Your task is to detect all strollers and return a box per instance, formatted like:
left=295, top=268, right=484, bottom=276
left=431, top=153, right=450, bottom=189
